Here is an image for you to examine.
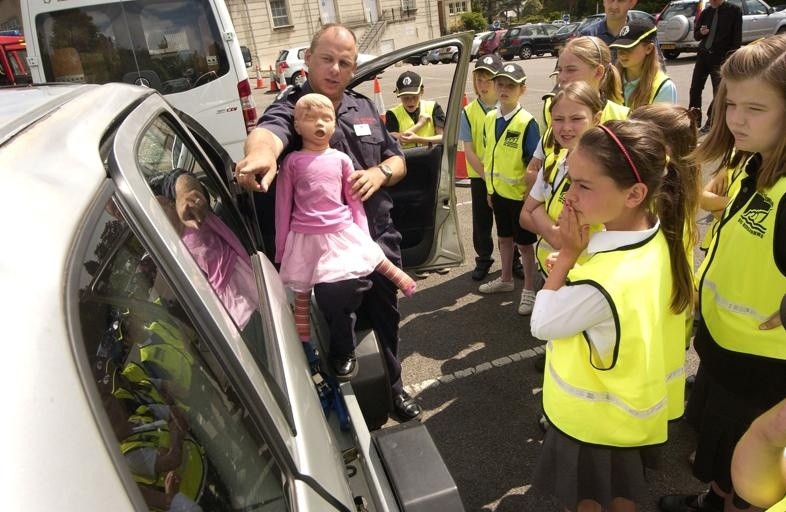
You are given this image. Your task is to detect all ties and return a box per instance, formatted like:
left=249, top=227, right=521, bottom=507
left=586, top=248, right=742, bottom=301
left=705, top=9, right=719, bottom=50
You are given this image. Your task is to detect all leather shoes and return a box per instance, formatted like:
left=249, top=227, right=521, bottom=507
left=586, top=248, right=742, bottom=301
left=658, top=490, right=725, bottom=512
left=392, top=390, right=424, bottom=424
left=327, top=348, right=359, bottom=383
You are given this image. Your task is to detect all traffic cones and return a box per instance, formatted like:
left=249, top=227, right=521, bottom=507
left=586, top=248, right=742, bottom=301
left=254, top=63, right=306, bottom=94
left=447, top=91, right=469, bottom=182
left=368, top=75, right=393, bottom=129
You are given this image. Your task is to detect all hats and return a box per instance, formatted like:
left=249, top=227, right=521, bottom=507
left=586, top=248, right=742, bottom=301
left=608, top=19, right=658, bottom=51
left=490, top=63, right=527, bottom=85
left=472, top=54, right=504, bottom=76
left=395, top=71, right=423, bottom=99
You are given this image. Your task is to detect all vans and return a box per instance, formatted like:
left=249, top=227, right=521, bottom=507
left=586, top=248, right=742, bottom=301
left=18, top=0, right=269, bottom=176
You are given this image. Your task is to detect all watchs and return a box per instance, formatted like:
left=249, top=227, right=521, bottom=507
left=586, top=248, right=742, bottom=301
left=375, top=161, right=395, bottom=183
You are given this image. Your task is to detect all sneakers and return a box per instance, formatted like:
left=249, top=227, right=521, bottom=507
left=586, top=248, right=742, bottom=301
left=472, top=266, right=490, bottom=281
left=518, top=288, right=537, bottom=316
left=512, top=262, right=525, bottom=280
left=478, top=276, right=515, bottom=293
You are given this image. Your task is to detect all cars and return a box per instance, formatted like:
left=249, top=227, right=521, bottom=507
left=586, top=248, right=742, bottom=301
left=0, top=27, right=483, bottom=512
left=579, top=3, right=664, bottom=60
left=403, top=25, right=505, bottom=70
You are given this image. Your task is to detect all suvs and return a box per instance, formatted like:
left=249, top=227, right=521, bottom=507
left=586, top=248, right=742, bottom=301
left=0, top=30, right=32, bottom=84
left=547, top=14, right=616, bottom=70
left=268, top=31, right=387, bottom=85
left=648, top=0, right=785, bottom=67
left=501, top=15, right=585, bottom=64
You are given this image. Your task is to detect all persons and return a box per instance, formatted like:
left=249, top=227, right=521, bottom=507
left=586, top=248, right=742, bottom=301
left=276, top=90, right=417, bottom=344
left=378, top=69, right=452, bottom=280
left=689, top=1, right=742, bottom=132
left=461, top=55, right=539, bottom=314
left=517, top=22, right=785, bottom=511
left=76, top=168, right=262, bottom=512
left=232, top=24, right=424, bottom=423
left=579, top=1, right=666, bottom=74
